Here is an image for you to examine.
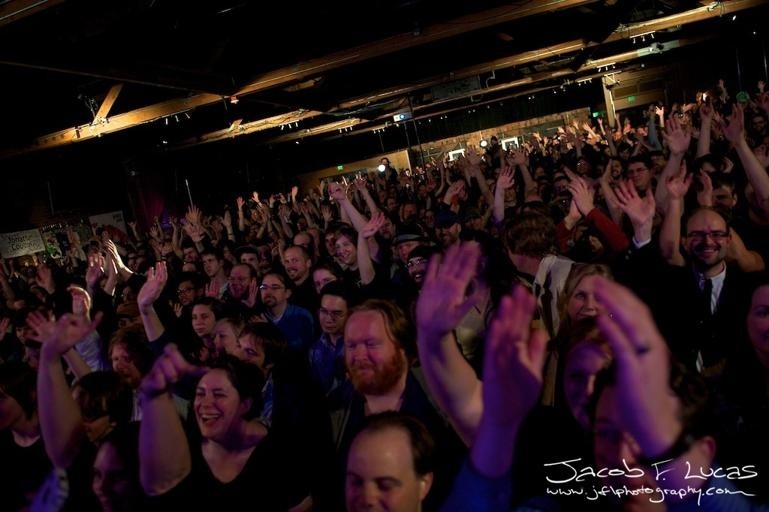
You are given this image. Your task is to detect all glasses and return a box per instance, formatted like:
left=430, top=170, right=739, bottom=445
left=320, top=308, right=348, bottom=319
left=686, top=229, right=729, bottom=242
left=260, top=285, right=285, bottom=290
left=177, top=287, right=192, bottom=295
left=407, top=258, right=427, bottom=268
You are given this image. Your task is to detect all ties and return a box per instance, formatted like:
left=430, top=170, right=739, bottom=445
left=694, top=281, right=713, bottom=380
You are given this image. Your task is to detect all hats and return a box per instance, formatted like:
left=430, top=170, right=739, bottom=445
left=434, top=211, right=458, bottom=230
left=392, top=224, right=425, bottom=246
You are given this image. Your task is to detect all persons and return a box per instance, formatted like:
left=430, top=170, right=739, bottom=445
left=0, top=79, right=769, bottom=512
